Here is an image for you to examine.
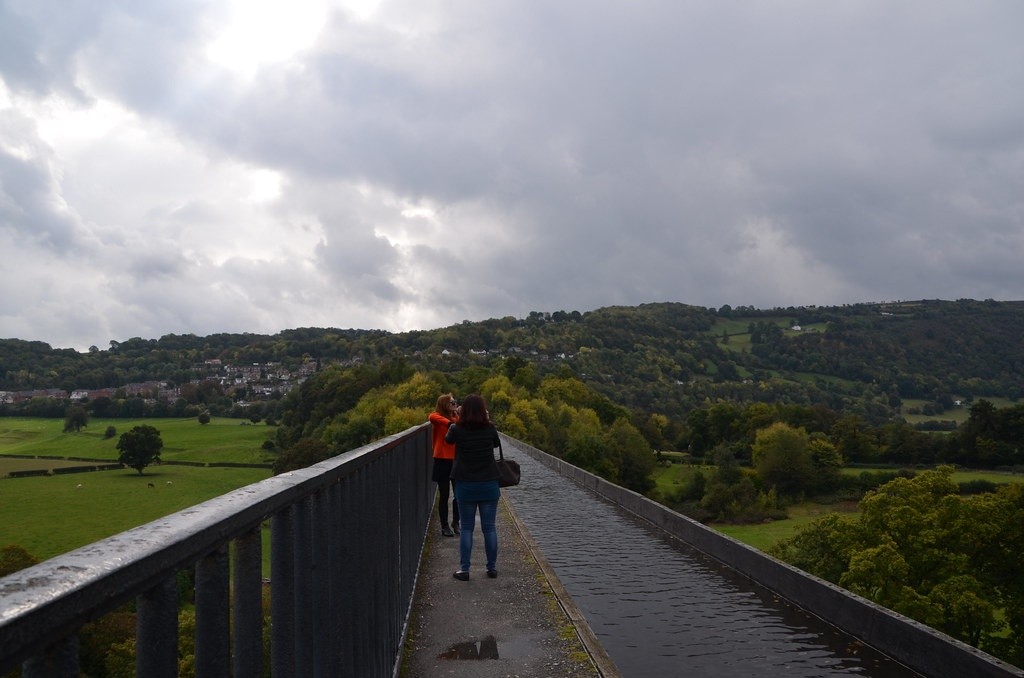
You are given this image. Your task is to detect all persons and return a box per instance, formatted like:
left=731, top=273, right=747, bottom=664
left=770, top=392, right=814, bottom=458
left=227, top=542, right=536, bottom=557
left=428, top=393, right=461, bottom=537
left=444, top=395, right=501, bottom=581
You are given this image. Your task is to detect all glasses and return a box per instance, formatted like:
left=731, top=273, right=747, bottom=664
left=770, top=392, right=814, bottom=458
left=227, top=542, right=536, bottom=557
left=449, top=400, right=457, bottom=406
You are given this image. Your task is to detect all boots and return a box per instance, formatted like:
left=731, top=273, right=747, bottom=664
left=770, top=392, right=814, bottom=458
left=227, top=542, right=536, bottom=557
left=438, top=501, right=454, bottom=537
left=451, top=499, right=461, bottom=534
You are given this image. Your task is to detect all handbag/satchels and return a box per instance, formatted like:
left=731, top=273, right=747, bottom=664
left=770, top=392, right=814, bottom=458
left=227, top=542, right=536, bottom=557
left=495, top=439, right=521, bottom=488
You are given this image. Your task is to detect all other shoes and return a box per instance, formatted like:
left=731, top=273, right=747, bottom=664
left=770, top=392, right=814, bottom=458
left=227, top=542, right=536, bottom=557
left=453, top=571, right=469, bottom=581
left=487, top=570, right=497, bottom=579
